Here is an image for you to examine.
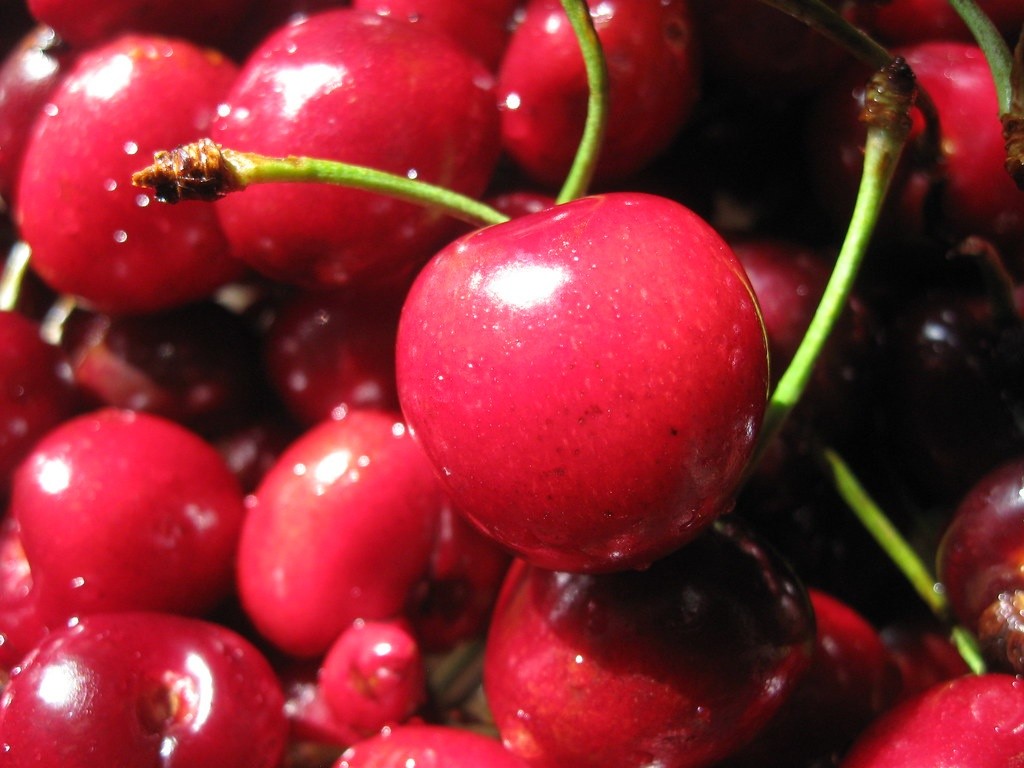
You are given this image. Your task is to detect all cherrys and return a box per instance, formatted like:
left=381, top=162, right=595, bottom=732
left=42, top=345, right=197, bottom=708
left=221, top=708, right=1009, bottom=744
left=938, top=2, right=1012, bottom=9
left=0, top=0, right=1024, bottom=768
